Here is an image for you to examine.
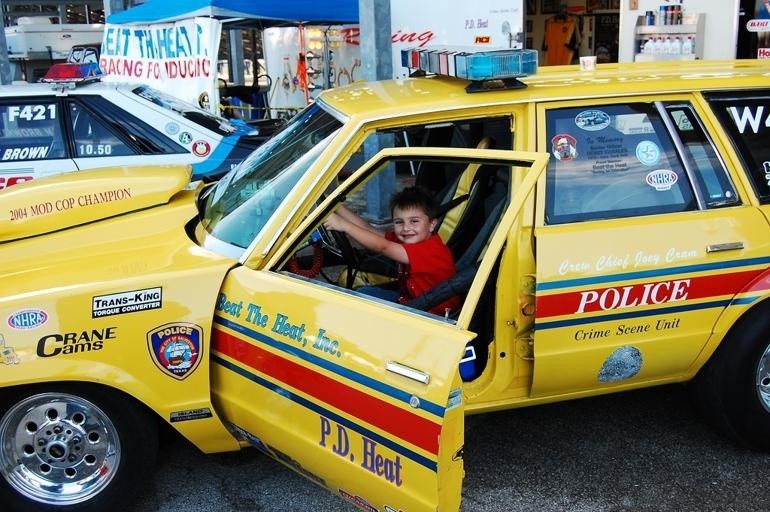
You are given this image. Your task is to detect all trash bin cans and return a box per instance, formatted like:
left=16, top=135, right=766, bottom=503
left=228, top=85, right=261, bottom=121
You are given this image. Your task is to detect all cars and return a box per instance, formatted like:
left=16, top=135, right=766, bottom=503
left=0, top=61, right=269, bottom=188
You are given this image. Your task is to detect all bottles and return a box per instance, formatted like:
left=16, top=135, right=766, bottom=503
left=282, top=51, right=335, bottom=92
left=640, top=0, right=696, bottom=57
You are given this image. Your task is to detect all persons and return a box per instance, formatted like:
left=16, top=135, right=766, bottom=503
left=322, top=183, right=461, bottom=316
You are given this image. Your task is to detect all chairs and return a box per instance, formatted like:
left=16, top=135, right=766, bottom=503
left=455, top=196, right=506, bottom=333
left=338, top=136, right=497, bottom=293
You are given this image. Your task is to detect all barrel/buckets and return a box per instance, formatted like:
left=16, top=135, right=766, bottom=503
left=643, top=35, right=695, bottom=56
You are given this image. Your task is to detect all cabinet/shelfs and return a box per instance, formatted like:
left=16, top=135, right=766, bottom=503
left=630, top=12, right=705, bottom=62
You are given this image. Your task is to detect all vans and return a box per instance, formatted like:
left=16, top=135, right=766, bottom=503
left=0, top=43, right=770, bottom=510
